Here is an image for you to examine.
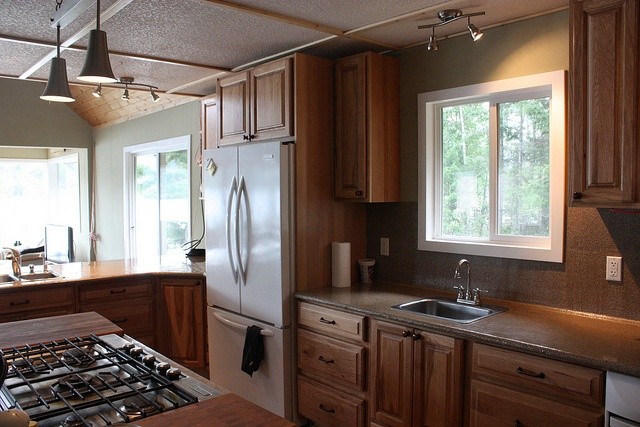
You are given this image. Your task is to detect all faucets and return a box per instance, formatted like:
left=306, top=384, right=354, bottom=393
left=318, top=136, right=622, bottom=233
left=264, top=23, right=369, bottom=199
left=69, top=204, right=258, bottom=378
left=453, top=258, right=473, bottom=302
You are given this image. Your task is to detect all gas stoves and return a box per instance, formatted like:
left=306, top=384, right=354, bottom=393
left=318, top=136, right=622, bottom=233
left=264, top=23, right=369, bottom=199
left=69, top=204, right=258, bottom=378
left=0, top=333, right=222, bottom=427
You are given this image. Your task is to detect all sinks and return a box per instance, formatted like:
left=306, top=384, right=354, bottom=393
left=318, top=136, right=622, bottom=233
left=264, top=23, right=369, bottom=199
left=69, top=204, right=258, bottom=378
left=400, top=300, right=493, bottom=324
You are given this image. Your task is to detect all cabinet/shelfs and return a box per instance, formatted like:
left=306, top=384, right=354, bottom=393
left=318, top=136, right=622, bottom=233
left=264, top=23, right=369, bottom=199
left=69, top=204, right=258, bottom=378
left=298, top=301, right=369, bottom=426
left=216, top=57, right=292, bottom=148
left=79, top=278, right=156, bottom=350
left=158, top=278, right=204, bottom=369
left=367, top=317, right=464, bottom=426
left=568, top=1, right=640, bottom=207
left=202, top=101, right=216, bottom=149
left=335, top=51, right=400, bottom=203
left=0, top=286, right=76, bottom=323
left=465, top=340, right=605, bottom=426
left=605, top=371, right=640, bottom=426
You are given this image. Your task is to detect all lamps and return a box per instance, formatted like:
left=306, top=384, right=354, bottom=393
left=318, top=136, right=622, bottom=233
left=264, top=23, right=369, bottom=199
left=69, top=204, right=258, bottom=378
left=418, top=9, right=485, bottom=51
left=92, top=76, right=160, bottom=102
left=76, top=0, right=119, bottom=86
left=40, top=24, right=75, bottom=102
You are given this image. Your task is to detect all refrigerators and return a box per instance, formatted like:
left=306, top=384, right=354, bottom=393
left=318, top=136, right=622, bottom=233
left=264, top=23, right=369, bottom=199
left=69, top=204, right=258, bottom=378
left=202, top=141, right=297, bottom=427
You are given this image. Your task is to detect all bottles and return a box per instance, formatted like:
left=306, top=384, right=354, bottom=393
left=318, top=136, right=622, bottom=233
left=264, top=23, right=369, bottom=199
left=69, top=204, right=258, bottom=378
left=359, top=259, right=376, bottom=284
